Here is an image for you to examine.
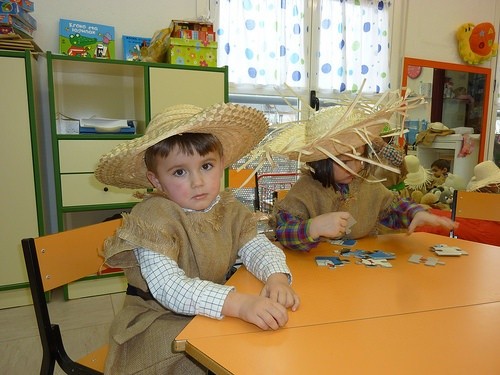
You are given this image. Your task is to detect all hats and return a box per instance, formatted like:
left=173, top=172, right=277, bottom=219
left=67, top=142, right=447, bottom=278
left=96, top=104, right=269, bottom=190
left=466, top=161, right=500, bottom=191
left=403, top=155, right=433, bottom=190
left=236, top=77, right=426, bottom=194
left=428, top=121, right=449, bottom=132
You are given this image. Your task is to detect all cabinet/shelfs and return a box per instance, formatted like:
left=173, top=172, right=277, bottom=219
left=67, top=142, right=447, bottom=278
left=0, top=49, right=52, bottom=310
left=46, top=47, right=230, bottom=301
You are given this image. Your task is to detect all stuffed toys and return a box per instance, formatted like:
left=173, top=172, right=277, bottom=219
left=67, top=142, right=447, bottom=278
left=411, top=184, right=454, bottom=210
left=456, top=22, right=498, bottom=64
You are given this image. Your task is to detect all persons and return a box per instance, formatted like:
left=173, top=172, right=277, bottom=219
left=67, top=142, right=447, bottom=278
left=467, top=161, right=500, bottom=194
left=267, top=105, right=460, bottom=252
left=95, top=102, right=300, bottom=375
left=431, top=159, right=452, bottom=186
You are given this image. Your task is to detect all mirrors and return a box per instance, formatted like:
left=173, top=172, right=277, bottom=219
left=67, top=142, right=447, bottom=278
left=399, top=57, right=493, bottom=211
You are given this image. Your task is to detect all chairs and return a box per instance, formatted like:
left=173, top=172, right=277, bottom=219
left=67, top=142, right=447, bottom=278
left=449, top=189, right=500, bottom=240
left=229, top=168, right=291, bottom=215
left=20, top=216, right=129, bottom=375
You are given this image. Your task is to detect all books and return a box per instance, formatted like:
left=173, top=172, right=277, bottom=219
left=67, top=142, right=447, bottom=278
left=122, top=36, right=152, bottom=61
left=59, top=19, right=115, bottom=59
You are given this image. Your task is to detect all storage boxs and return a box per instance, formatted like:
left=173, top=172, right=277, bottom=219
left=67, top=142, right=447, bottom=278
left=121, top=34, right=152, bottom=61
left=0, top=0, right=38, bottom=36
left=164, top=20, right=218, bottom=68
left=56, top=118, right=80, bottom=135
left=58, top=18, right=116, bottom=60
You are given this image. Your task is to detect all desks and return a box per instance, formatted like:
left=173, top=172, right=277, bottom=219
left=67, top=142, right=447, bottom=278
left=174, top=230, right=500, bottom=375
left=415, top=133, right=481, bottom=187
